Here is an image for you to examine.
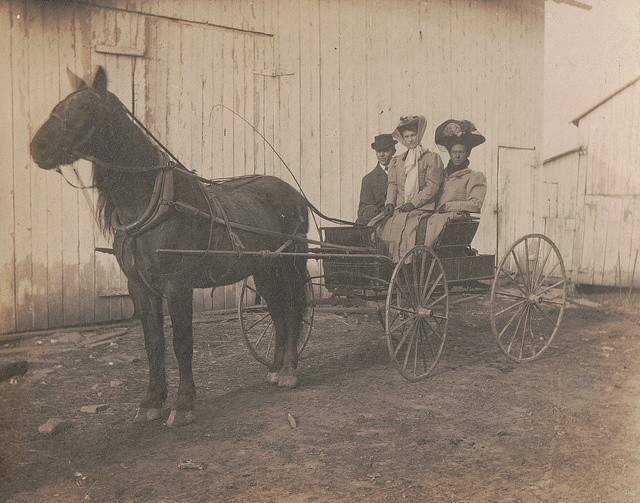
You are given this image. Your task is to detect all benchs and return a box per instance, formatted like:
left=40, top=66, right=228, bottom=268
left=355, top=211, right=479, bottom=251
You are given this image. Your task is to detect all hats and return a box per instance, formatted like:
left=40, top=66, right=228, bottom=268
left=435, top=119, right=486, bottom=147
left=371, top=133, right=398, bottom=150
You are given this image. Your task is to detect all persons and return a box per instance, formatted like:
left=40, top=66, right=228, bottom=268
left=367, top=116, right=444, bottom=265
left=417, top=119, right=487, bottom=257
left=358, top=134, right=399, bottom=226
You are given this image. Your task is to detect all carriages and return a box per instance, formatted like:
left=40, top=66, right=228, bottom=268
left=29, top=64, right=568, bottom=428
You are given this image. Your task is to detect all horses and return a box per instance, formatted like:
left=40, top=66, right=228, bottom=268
left=29, top=66, right=309, bottom=427
